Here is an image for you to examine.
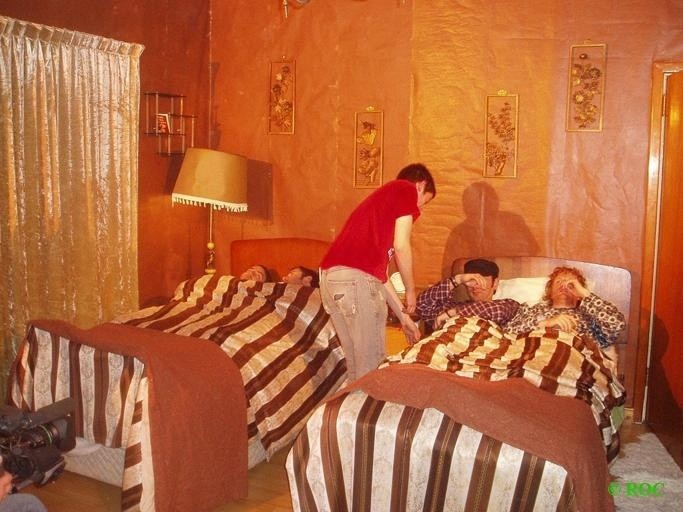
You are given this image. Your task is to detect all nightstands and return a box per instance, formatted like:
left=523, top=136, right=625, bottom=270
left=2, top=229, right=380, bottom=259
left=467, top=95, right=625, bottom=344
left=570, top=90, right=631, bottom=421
left=384, top=314, right=425, bottom=358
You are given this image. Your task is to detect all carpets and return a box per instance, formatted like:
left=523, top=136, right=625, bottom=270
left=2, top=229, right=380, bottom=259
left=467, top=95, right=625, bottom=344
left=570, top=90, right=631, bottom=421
left=607, top=414, right=681, bottom=512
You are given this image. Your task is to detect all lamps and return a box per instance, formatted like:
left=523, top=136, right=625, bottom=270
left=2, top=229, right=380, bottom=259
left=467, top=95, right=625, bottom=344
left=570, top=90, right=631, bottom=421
left=169, top=146, right=248, bottom=276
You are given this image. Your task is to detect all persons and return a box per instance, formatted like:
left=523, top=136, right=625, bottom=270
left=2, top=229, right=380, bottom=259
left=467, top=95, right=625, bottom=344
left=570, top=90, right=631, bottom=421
left=416, top=260, right=518, bottom=331
left=0, top=454, right=47, bottom=512
left=321, top=163, right=436, bottom=385
left=507, top=266, right=626, bottom=349
left=239, top=264, right=271, bottom=283
left=281, top=266, right=320, bottom=288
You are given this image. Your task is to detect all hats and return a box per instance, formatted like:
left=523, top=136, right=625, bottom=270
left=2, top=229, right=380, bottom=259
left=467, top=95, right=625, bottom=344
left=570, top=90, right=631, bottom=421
left=463, top=258, right=499, bottom=276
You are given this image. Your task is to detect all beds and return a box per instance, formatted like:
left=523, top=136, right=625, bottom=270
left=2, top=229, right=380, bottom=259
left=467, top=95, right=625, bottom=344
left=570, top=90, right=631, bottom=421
left=5, top=235, right=354, bottom=512
left=283, top=255, right=632, bottom=512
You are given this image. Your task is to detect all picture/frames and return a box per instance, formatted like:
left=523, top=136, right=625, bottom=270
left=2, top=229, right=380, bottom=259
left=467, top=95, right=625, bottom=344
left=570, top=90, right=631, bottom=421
left=565, top=43, right=607, bottom=133
left=482, top=92, right=519, bottom=179
left=266, top=58, right=296, bottom=135
left=353, top=109, right=384, bottom=190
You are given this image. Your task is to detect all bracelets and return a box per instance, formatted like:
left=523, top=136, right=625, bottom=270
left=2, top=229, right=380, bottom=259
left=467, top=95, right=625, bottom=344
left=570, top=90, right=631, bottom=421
left=445, top=310, right=454, bottom=321
left=450, top=277, right=460, bottom=289
